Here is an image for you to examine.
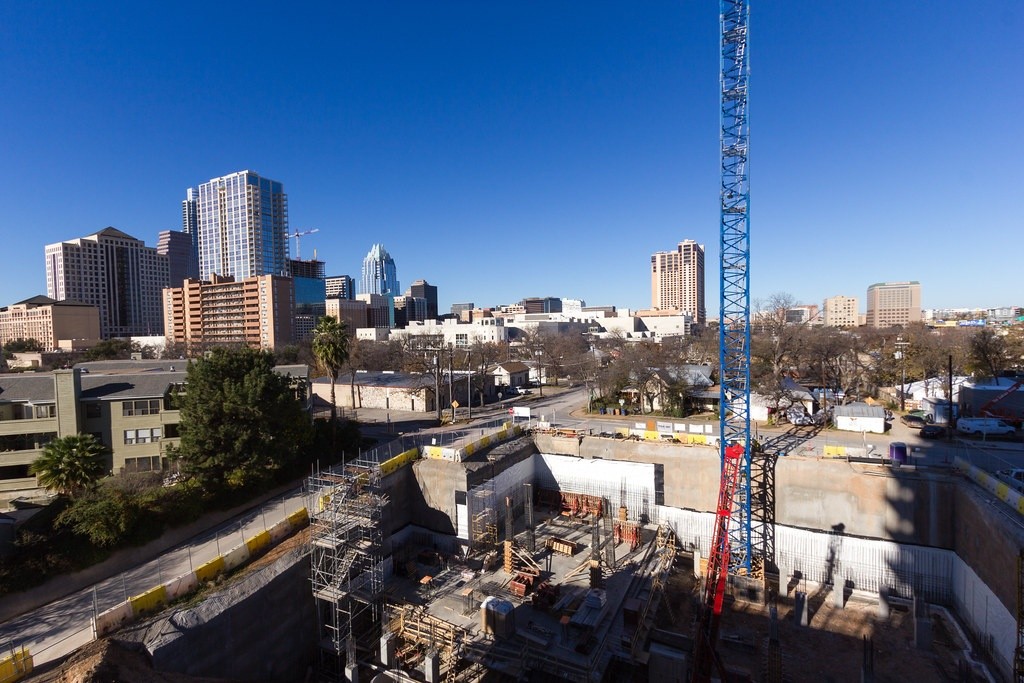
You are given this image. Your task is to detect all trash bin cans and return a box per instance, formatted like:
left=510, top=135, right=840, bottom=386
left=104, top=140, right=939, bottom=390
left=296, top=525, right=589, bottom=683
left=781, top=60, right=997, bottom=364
left=599, top=407, right=605, bottom=415
left=607, top=408, right=614, bottom=415
left=620, top=408, right=627, bottom=416
left=615, top=408, right=621, bottom=416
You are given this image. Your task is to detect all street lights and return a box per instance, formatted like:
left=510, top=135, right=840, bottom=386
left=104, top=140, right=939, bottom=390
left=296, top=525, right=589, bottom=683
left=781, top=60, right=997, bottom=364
left=895, top=335, right=907, bottom=412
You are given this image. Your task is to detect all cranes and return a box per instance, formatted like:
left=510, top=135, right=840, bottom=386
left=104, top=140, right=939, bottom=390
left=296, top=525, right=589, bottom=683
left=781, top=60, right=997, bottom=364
left=684, top=442, right=754, bottom=683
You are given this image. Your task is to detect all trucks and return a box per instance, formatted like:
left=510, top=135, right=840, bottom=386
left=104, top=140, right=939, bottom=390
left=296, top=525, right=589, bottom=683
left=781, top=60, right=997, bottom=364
left=956, top=418, right=1017, bottom=437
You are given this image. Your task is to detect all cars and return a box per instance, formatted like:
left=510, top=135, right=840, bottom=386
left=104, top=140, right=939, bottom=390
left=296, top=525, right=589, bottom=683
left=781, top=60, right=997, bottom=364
left=900, top=415, right=928, bottom=429
left=995, top=468, right=1024, bottom=497
left=921, top=425, right=948, bottom=440
